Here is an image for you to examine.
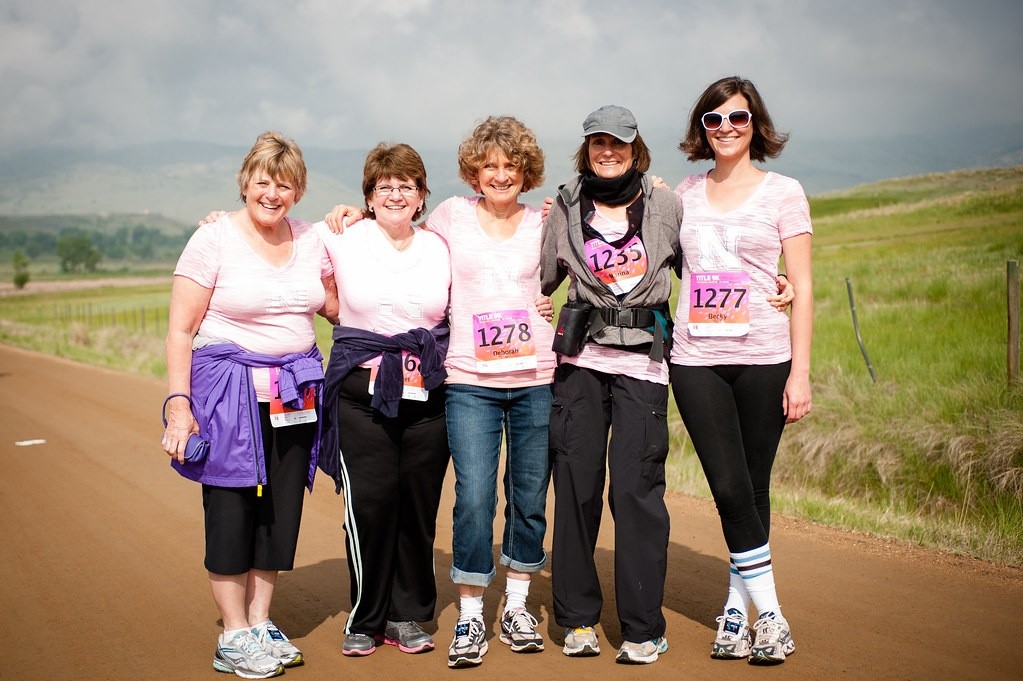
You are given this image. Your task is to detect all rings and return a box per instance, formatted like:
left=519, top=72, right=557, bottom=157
left=166, top=441, right=171, bottom=444
left=552, top=313, right=554, bottom=317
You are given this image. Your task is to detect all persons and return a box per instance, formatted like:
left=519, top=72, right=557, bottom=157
left=197, top=143, right=555, bottom=657
left=162, top=133, right=338, bottom=679
left=325, top=116, right=670, bottom=668
left=541, top=104, right=794, bottom=665
left=541, top=77, right=813, bottom=664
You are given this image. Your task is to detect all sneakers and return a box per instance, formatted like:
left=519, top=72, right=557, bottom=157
left=381, top=620, right=435, bottom=653
left=212, top=630, right=285, bottom=678
left=342, top=632, right=381, bottom=656
left=710, top=606, right=753, bottom=659
left=616, top=633, right=669, bottom=664
left=747, top=605, right=795, bottom=664
left=562, top=625, right=601, bottom=656
left=250, top=621, right=304, bottom=668
left=499, top=606, right=545, bottom=653
left=447, top=614, right=489, bottom=668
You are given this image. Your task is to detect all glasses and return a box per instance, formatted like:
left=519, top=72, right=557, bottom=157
left=700, top=110, right=752, bottom=131
left=371, top=184, right=422, bottom=197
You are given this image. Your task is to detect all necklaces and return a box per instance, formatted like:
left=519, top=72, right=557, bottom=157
left=381, top=229, right=413, bottom=250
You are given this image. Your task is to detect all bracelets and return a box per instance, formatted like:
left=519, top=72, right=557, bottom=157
left=778, top=274, right=787, bottom=279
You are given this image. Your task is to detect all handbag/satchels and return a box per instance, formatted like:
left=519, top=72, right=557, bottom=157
left=162, top=394, right=210, bottom=464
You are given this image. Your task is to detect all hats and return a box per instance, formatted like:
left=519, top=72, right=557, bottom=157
left=581, top=105, right=638, bottom=144
